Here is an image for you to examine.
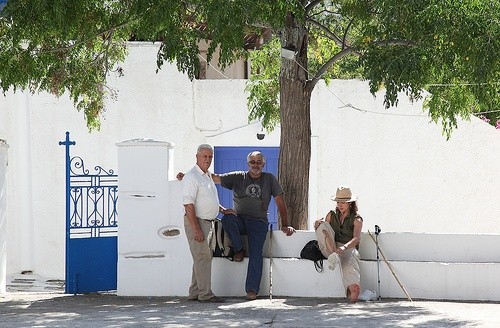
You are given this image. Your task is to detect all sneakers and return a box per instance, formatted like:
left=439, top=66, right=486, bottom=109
left=199, top=296, right=224, bottom=303
left=233, top=248, right=245, bottom=262
left=189, top=298, right=198, bottom=301
left=357, top=290, right=377, bottom=300
left=328, top=252, right=338, bottom=271
left=247, top=291, right=256, bottom=299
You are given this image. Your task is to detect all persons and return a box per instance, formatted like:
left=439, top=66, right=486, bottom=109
left=314, top=187, right=363, bottom=303
left=181, top=144, right=239, bottom=303
left=176, top=152, right=296, bottom=300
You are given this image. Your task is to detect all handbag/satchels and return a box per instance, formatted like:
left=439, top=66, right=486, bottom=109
left=300, top=240, right=327, bottom=273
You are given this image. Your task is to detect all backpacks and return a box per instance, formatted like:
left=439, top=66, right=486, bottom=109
left=209, top=219, right=233, bottom=261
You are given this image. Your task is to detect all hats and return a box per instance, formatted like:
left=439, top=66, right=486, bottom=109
left=331, top=186, right=357, bottom=202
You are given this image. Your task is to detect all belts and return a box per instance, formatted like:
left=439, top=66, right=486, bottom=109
left=239, top=216, right=268, bottom=224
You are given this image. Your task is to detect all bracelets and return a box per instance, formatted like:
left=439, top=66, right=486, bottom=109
left=343, top=245, right=347, bottom=249
left=221, top=208, right=225, bottom=213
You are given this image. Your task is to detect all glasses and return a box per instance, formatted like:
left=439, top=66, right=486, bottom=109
left=248, top=160, right=263, bottom=164
